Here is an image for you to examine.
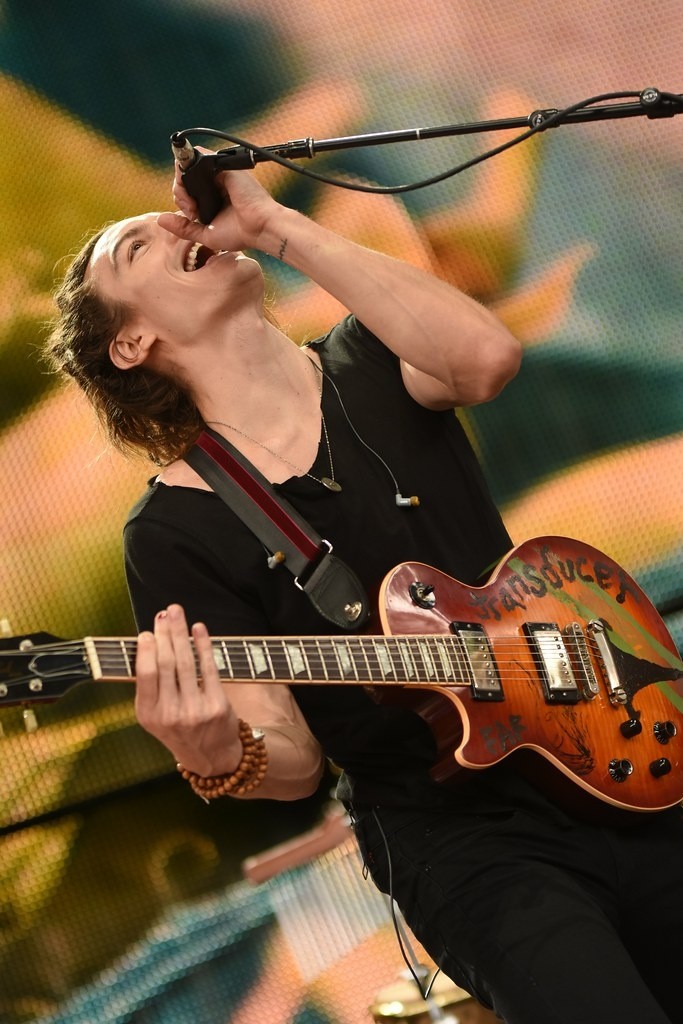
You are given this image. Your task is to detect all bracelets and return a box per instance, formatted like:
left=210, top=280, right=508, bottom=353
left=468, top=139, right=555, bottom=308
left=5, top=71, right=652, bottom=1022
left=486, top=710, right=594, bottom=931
left=177, top=717, right=269, bottom=804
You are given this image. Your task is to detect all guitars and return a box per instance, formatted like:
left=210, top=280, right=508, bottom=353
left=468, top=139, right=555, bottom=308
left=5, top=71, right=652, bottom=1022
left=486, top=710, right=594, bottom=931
left=1, top=534, right=683, bottom=812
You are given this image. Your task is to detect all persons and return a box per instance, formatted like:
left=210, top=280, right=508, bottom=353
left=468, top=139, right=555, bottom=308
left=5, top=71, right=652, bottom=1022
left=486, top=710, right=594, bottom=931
left=29, top=145, right=683, bottom=1024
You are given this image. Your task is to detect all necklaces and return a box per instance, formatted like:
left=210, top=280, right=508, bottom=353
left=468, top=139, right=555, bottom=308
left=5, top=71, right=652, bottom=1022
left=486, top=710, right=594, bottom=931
left=204, top=356, right=341, bottom=491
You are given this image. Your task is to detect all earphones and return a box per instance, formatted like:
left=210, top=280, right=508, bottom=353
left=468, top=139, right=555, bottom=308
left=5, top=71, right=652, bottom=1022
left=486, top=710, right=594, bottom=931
left=395, top=493, right=421, bottom=506
left=266, top=552, right=285, bottom=569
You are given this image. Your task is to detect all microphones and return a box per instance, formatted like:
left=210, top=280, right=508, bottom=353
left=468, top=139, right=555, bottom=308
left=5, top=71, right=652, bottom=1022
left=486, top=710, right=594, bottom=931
left=171, top=133, right=225, bottom=226
